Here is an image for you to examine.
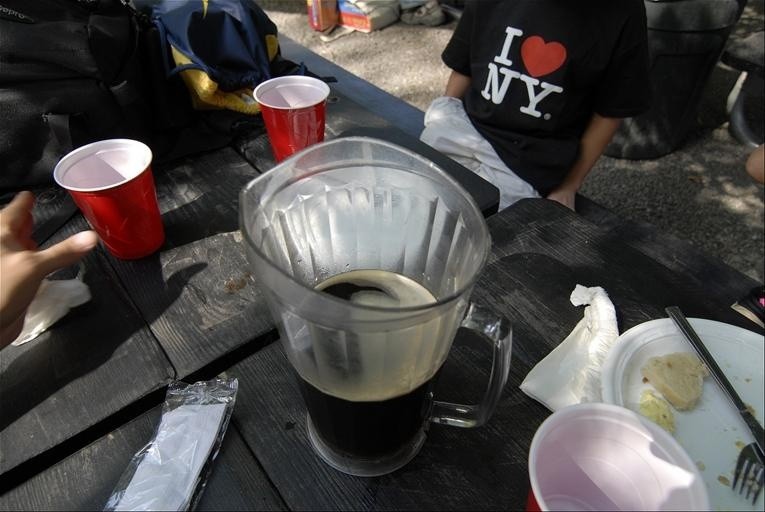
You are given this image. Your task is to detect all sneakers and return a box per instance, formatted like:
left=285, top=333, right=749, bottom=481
left=398, top=1, right=448, bottom=28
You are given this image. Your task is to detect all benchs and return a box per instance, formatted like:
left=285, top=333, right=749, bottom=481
left=278, top=33, right=764, bottom=313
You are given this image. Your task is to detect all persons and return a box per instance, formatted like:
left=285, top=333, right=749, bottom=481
left=0, top=190, right=97, bottom=352
left=420, top=0, right=648, bottom=213
left=745, top=144, right=765, bottom=185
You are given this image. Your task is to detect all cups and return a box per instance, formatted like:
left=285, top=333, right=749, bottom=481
left=252, top=75, right=331, bottom=167
left=237, top=137, right=517, bottom=478
left=527, top=403, right=710, bottom=511
left=53, top=138, right=165, bottom=262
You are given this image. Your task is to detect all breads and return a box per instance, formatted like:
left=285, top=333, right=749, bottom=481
left=639, top=352, right=710, bottom=435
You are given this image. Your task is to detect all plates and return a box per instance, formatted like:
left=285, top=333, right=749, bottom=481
left=602, top=318, right=765, bottom=511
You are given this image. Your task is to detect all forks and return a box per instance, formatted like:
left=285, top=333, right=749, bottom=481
left=732, top=440, right=765, bottom=505
